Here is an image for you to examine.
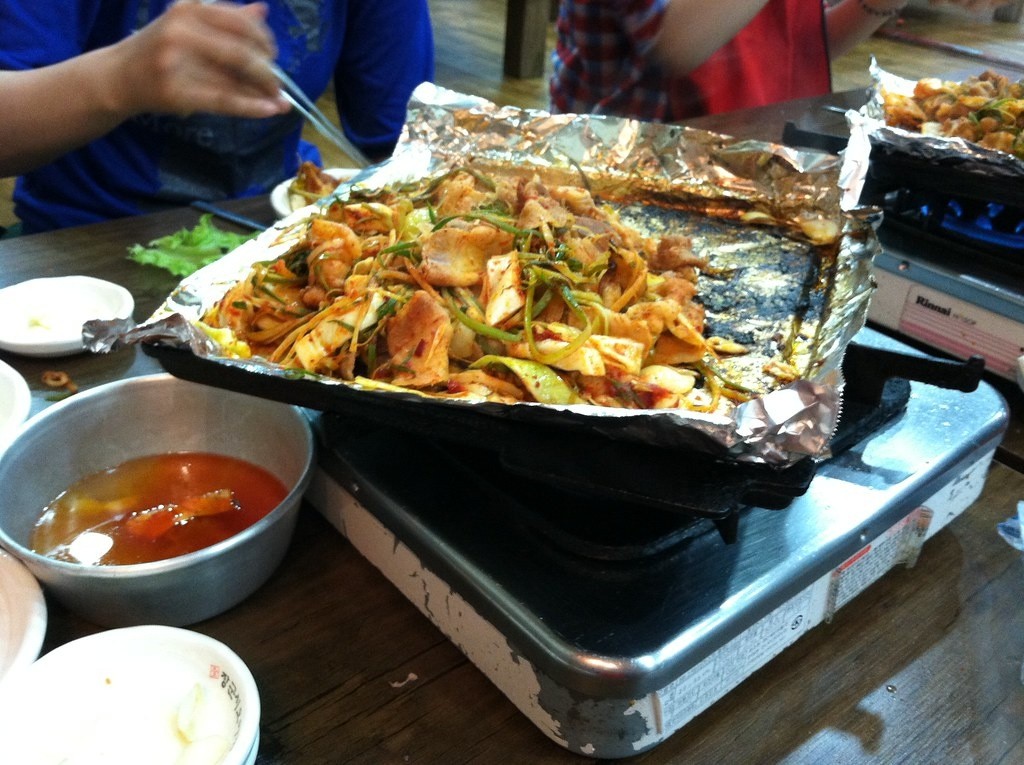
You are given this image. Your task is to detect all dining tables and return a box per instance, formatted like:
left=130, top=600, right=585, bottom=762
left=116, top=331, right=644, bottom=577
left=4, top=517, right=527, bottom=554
left=668, top=64, right=1024, bottom=473
left=0, top=192, right=1024, bottom=765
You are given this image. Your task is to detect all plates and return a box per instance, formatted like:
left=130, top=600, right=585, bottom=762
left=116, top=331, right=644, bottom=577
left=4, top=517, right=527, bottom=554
left=0, top=276, right=135, bottom=358
left=0, top=624, right=261, bottom=765
left=0, top=549, right=47, bottom=683
left=270, top=168, right=362, bottom=218
left=0, top=360, right=31, bottom=440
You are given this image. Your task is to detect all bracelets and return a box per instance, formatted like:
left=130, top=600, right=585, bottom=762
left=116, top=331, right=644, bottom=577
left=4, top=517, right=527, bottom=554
left=857, top=1, right=906, bottom=16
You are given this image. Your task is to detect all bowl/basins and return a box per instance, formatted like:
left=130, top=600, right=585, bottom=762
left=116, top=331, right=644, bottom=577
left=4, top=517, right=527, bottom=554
left=1, top=372, right=270, bottom=628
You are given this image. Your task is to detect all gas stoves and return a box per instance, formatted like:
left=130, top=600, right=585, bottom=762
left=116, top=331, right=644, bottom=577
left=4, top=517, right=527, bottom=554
left=288, top=321, right=1007, bottom=757
left=868, top=173, right=1024, bottom=396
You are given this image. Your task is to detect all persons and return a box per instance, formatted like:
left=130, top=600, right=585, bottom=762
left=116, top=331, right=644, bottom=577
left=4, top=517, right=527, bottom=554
left=0, top=0, right=437, bottom=230
left=549, top=0, right=914, bottom=128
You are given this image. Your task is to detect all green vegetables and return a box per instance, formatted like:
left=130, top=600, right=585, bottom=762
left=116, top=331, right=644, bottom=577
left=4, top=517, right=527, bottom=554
left=233, top=166, right=594, bottom=364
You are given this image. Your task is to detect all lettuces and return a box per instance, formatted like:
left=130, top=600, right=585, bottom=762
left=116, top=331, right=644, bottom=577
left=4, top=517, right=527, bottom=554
left=125, top=213, right=264, bottom=278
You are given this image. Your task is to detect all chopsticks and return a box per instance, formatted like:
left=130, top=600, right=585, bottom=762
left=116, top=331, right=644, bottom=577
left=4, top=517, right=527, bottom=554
left=267, top=59, right=373, bottom=171
